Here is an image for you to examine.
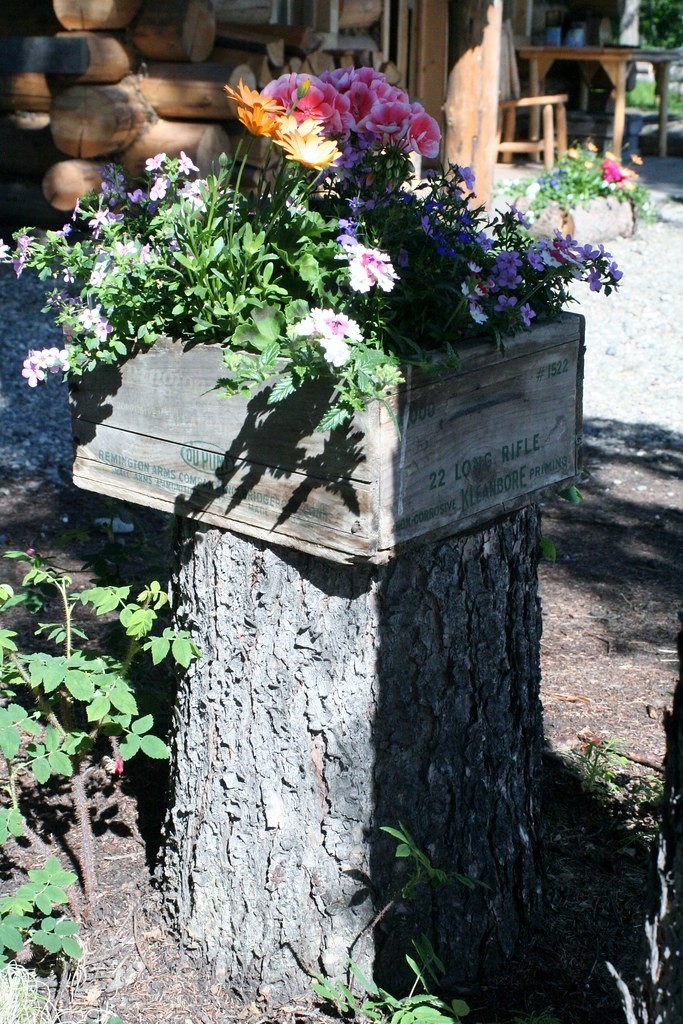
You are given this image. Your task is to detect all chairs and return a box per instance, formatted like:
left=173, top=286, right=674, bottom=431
left=497, top=20, right=567, bottom=169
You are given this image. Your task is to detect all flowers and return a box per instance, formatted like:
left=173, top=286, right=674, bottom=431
left=530, top=141, right=646, bottom=210
left=2, top=67, right=650, bottom=432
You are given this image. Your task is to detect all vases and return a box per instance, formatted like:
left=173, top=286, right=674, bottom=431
left=513, top=194, right=636, bottom=239
left=62, top=313, right=587, bottom=567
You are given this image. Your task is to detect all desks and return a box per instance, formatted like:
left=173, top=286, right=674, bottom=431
left=515, top=42, right=682, bottom=162
